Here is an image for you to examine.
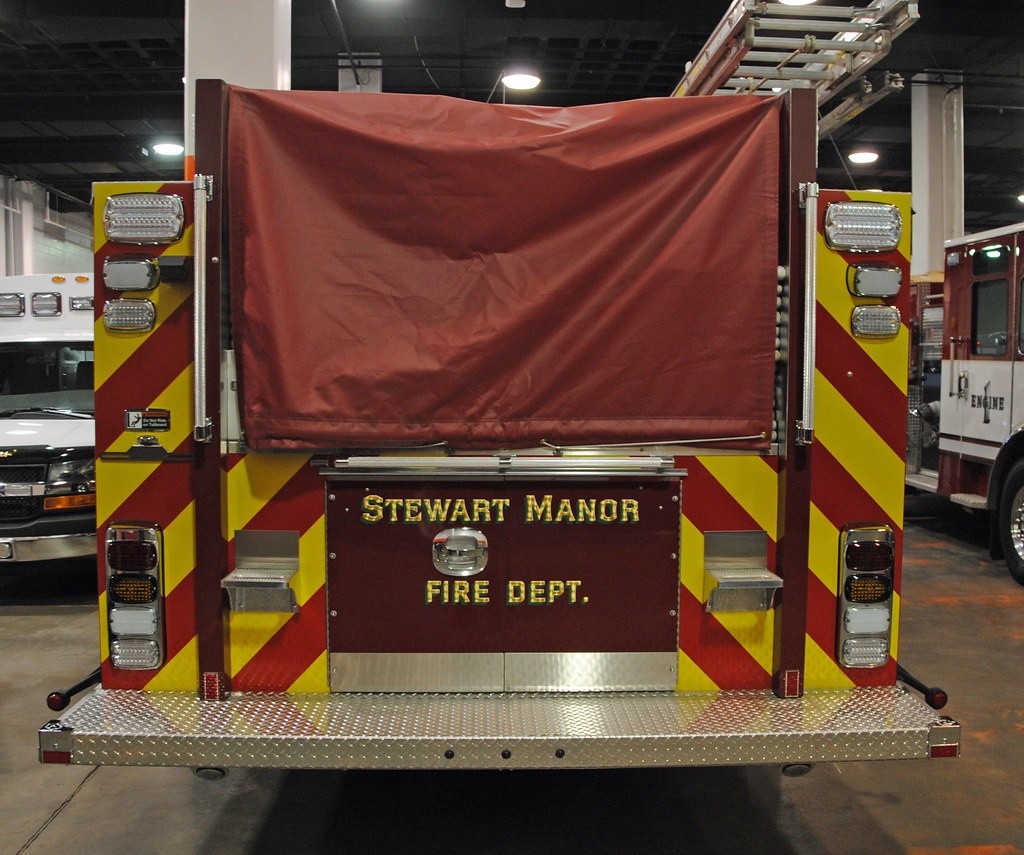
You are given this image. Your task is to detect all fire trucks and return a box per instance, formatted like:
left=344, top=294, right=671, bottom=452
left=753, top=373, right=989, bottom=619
left=37, top=0, right=961, bottom=769
left=905, top=221, right=1024, bottom=586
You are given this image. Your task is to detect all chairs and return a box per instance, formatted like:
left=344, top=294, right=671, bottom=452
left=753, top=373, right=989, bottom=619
left=2, top=360, right=45, bottom=394
left=75, top=361, right=93, bottom=390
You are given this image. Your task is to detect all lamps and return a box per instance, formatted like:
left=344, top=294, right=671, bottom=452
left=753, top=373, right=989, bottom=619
left=501, top=63, right=542, bottom=89
left=848, top=144, right=880, bottom=163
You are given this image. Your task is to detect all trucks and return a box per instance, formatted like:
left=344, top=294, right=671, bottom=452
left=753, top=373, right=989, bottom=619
left=0, top=273, right=98, bottom=562
